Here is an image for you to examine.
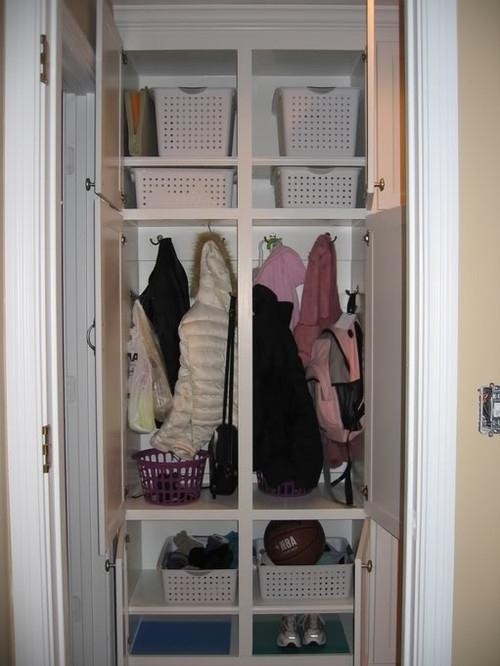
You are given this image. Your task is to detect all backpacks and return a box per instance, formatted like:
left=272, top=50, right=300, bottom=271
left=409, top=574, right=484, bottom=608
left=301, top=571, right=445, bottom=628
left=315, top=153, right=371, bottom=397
left=306, top=313, right=366, bottom=443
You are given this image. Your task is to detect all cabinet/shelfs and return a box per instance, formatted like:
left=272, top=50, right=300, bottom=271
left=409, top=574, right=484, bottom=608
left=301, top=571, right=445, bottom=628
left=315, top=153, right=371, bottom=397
left=86, top=5, right=408, bottom=666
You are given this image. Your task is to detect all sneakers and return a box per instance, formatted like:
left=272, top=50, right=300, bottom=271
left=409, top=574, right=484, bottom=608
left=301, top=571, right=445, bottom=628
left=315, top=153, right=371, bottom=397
left=277, top=614, right=302, bottom=649
left=302, top=614, right=327, bottom=649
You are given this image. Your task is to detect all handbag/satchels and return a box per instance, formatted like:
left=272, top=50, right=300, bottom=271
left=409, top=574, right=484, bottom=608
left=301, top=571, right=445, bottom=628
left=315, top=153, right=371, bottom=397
left=209, top=424, right=238, bottom=498
left=124, top=87, right=157, bottom=156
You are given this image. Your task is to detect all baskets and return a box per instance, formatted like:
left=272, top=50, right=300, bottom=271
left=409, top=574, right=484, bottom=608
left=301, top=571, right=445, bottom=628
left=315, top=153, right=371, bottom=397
left=150, top=87, right=236, bottom=157
left=131, top=447, right=208, bottom=505
left=271, top=87, right=359, bottom=157
left=157, top=536, right=238, bottom=603
left=254, top=537, right=353, bottom=599
left=130, top=168, right=236, bottom=208
left=269, top=166, right=361, bottom=209
left=257, top=472, right=312, bottom=496
